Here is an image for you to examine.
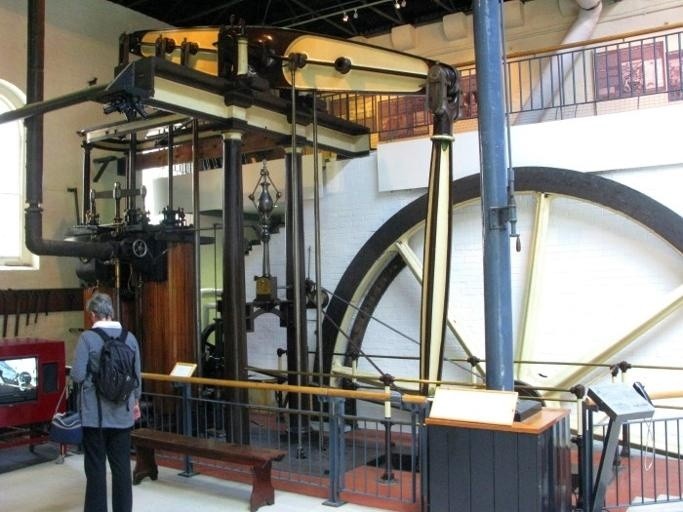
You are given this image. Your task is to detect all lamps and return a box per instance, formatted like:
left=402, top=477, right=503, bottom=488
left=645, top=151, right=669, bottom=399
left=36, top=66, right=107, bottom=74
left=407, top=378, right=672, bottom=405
left=341, top=0, right=406, bottom=23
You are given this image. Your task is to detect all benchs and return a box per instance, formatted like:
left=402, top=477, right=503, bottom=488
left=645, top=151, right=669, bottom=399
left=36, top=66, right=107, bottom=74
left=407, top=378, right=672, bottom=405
left=126, top=425, right=287, bottom=510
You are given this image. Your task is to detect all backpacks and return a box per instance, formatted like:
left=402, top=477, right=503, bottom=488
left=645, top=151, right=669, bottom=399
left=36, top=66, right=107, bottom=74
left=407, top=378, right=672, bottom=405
left=88, top=328, right=135, bottom=401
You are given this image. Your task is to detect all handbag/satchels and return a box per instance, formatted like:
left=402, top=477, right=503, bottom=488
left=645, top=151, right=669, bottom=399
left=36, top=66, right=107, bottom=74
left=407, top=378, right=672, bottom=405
left=49, top=411, right=83, bottom=444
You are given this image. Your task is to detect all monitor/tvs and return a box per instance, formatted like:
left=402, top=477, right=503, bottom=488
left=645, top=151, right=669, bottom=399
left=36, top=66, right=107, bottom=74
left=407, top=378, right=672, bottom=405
left=0, top=355, right=38, bottom=407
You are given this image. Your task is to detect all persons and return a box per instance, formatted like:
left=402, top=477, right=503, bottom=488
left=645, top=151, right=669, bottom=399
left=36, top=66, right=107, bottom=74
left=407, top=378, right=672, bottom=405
left=66, top=294, right=142, bottom=512
